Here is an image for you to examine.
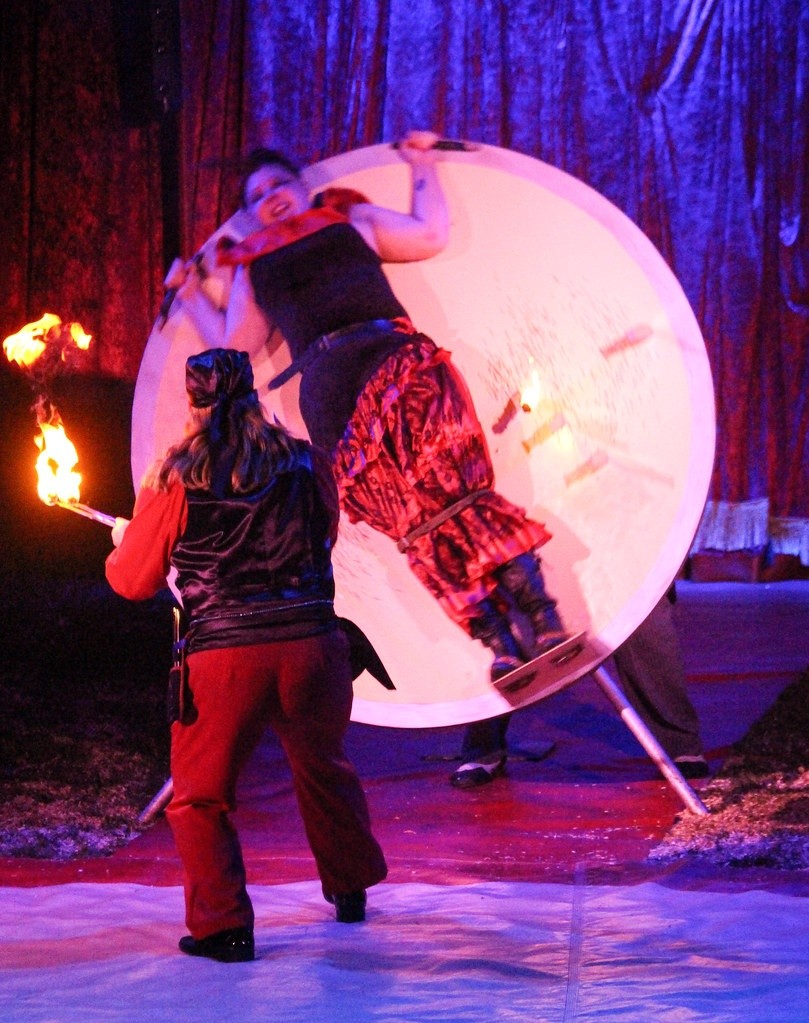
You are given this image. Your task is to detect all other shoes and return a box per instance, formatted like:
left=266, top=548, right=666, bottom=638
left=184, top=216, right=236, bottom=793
left=675, top=755, right=712, bottom=786
left=450, top=755, right=506, bottom=788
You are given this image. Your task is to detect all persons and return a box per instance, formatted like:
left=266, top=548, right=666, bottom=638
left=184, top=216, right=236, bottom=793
left=105, top=350, right=399, bottom=962
left=453, top=582, right=715, bottom=790
left=165, top=130, right=582, bottom=692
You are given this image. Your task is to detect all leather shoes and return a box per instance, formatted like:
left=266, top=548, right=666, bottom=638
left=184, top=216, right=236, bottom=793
left=333, top=890, right=367, bottom=922
left=179, top=927, right=254, bottom=962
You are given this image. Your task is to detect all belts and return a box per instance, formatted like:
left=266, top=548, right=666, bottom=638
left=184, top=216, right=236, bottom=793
left=265, top=320, right=390, bottom=390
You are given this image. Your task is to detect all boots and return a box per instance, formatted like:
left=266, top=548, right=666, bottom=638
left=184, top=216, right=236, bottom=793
left=468, top=596, right=534, bottom=692
left=492, top=552, right=578, bottom=665
